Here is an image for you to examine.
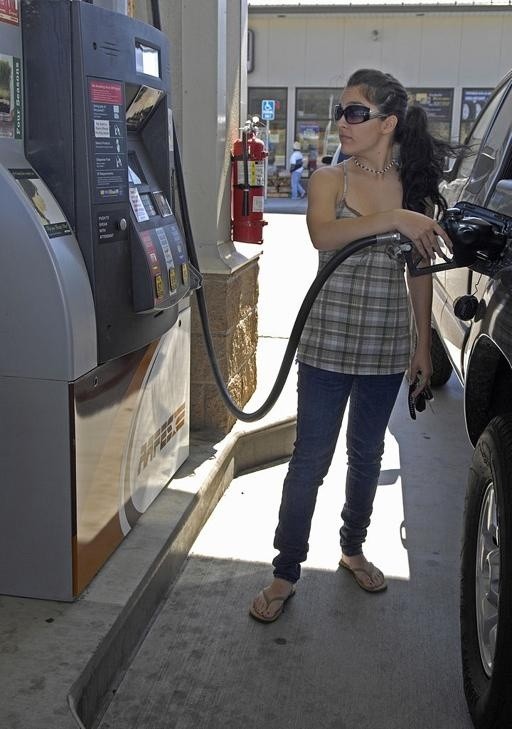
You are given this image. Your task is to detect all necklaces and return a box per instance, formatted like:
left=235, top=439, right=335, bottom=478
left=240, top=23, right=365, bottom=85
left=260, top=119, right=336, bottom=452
left=354, top=155, right=395, bottom=176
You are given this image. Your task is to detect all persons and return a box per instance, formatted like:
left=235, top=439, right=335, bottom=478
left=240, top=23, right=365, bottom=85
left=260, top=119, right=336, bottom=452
left=248, top=68, right=455, bottom=624
left=290, top=140, right=306, bottom=200
left=18, top=178, right=52, bottom=226
left=307, top=143, right=317, bottom=170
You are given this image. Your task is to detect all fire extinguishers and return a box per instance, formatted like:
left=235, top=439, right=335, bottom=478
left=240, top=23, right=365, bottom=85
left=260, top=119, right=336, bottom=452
left=232, top=120, right=268, bottom=244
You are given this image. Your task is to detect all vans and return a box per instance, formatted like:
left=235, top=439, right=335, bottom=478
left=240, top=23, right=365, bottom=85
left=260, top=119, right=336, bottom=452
left=319, top=144, right=351, bottom=166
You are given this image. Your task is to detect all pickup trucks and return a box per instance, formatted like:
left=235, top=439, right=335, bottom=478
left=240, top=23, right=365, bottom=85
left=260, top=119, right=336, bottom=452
left=415, top=67, right=511, bottom=727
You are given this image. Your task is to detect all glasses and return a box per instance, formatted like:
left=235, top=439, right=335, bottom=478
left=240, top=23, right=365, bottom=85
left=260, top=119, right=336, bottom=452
left=333, top=103, right=389, bottom=125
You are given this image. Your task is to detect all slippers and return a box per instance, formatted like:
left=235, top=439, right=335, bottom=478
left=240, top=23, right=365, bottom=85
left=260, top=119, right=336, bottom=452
left=248, top=582, right=297, bottom=623
left=338, top=556, right=389, bottom=593
